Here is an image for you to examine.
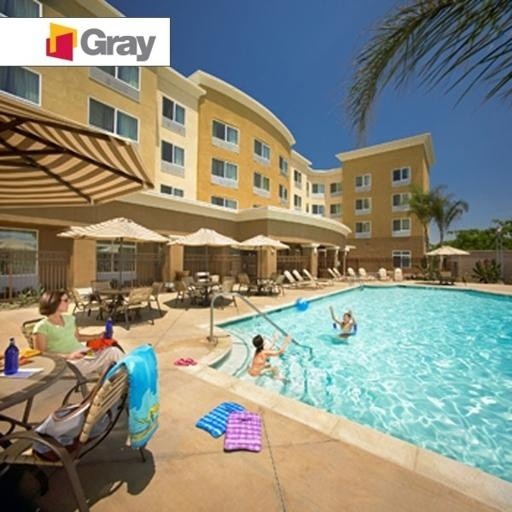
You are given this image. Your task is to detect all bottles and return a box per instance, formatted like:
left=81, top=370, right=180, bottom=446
left=104, top=317, right=113, bottom=338
left=4, top=337, right=19, bottom=374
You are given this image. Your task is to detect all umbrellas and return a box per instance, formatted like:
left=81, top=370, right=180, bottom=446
left=57, top=217, right=169, bottom=286
left=167, top=227, right=238, bottom=286
left=424, top=245, right=470, bottom=257
left=1, top=87, right=154, bottom=209
left=232, top=235, right=290, bottom=278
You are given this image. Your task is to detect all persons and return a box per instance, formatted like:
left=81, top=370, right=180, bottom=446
left=34, top=290, right=124, bottom=378
left=249, top=331, right=291, bottom=380
left=329, top=305, right=356, bottom=336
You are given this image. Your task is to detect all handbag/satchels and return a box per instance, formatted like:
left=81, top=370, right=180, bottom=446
left=34, top=387, right=93, bottom=455
